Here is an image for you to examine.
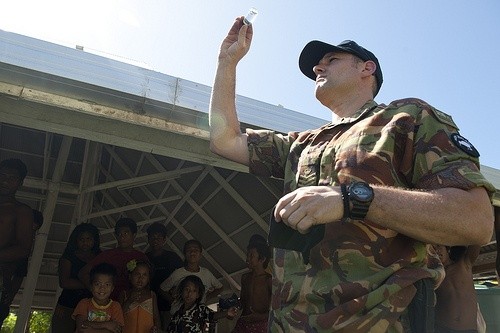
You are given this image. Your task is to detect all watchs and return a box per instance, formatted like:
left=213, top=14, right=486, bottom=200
left=347, top=181, right=375, bottom=221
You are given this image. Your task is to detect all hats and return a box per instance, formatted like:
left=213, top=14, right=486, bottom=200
left=299, top=40, right=383, bottom=97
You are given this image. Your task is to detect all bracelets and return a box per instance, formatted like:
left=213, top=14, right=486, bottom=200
left=341, top=183, right=350, bottom=218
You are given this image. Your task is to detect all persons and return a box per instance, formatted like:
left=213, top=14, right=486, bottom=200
left=231, top=234, right=274, bottom=333
left=208, top=16, right=495, bottom=333
left=0, top=158, right=43, bottom=325
left=432, top=243, right=487, bottom=333
left=51, top=217, right=239, bottom=332
